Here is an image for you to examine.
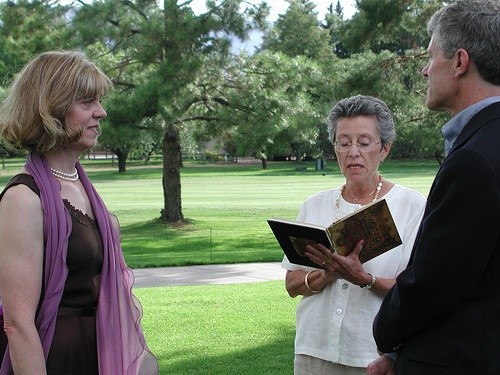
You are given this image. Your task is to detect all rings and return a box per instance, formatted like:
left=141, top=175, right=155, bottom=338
left=322, top=261, right=327, bottom=267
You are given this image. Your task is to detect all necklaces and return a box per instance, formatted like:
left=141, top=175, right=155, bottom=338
left=50, top=168, right=78, bottom=181
left=343, top=190, right=375, bottom=202
left=336, top=174, right=383, bottom=220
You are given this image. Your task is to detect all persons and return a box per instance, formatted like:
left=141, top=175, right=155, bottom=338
left=366, top=0, right=500, bottom=374
left=282, top=96, right=427, bottom=375
left=0, top=52, right=158, bottom=375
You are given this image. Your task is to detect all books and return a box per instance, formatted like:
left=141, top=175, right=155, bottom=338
left=268, top=199, right=403, bottom=268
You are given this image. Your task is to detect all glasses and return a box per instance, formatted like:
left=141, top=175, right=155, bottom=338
left=331, top=139, right=384, bottom=152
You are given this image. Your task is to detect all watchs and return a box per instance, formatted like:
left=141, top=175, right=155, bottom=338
left=360, top=273, right=376, bottom=289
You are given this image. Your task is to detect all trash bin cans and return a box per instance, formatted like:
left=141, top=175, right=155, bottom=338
left=315, top=159, right=326, bottom=170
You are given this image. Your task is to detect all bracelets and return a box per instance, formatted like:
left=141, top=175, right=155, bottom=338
left=304, top=270, right=320, bottom=293
